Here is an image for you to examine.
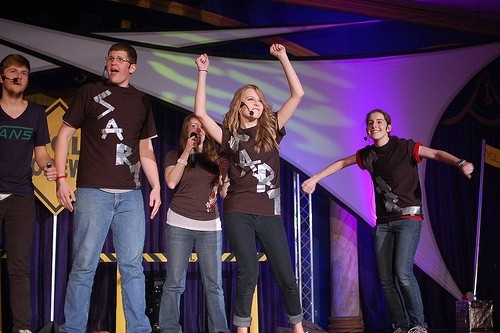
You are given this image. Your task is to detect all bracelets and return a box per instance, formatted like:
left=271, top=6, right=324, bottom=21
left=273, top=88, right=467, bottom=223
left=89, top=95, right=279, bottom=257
left=177, top=159, right=188, bottom=166
left=56, top=176, right=66, bottom=181
left=198, top=69, right=208, bottom=72
left=224, top=181, right=229, bottom=183
left=457, top=160, right=465, bottom=166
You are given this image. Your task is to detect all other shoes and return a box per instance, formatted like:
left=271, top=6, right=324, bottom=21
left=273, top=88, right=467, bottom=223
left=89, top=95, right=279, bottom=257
left=392, top=326, right=428, bottom=333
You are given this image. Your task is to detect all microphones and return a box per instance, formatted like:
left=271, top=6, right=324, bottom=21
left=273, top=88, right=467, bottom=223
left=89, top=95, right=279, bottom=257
left=364, top=137, right=367, bottom=141
left=1, top=76, right=18, bottom=82
left=101, top=69, right=106, bottom=79
left=244, top=103, right=254, bottom=115
left=190, top=132, right=197, bottom=163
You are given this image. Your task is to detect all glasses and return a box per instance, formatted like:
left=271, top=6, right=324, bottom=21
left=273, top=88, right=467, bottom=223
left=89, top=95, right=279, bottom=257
left=105, top=56, right=132, bottom=64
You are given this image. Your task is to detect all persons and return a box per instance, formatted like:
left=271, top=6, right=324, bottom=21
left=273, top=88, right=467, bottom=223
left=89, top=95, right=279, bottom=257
left=55, top=43, right=162, bottom=333
left=0, top=53, right=58, bottom=333
left=195, top=43, right=305, bottom=333
left=301, top=109, right=474, bottom=333
left=159, top=115, right=230, bottom=333
